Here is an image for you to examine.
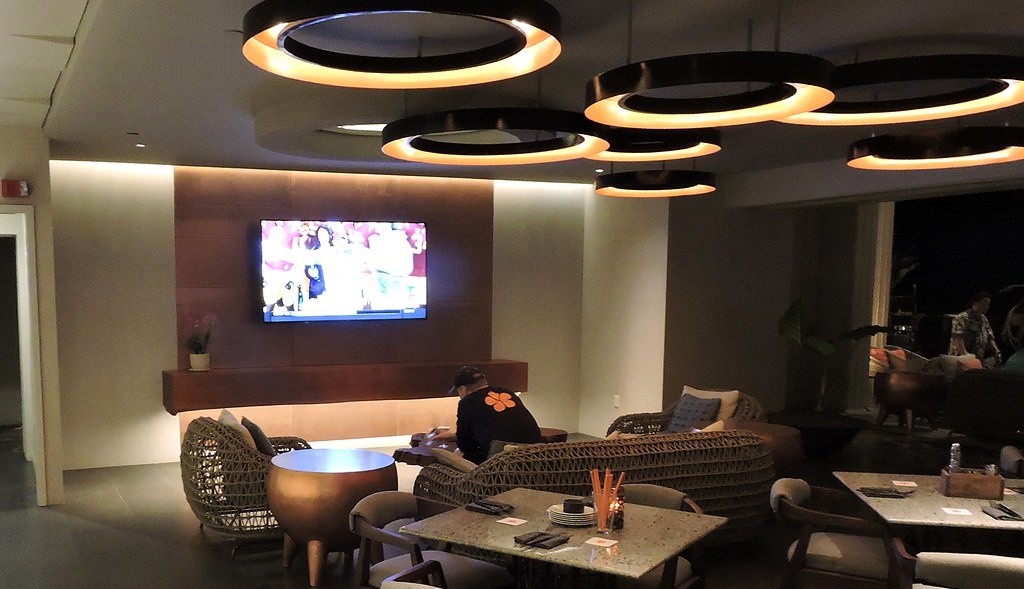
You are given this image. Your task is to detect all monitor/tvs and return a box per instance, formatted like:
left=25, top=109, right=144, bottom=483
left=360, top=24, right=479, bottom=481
left=259, top=218, right=428, bottom=321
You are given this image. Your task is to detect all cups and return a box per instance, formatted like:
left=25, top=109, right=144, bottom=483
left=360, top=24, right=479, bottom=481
left=592, top=488, right=616, bottom=535
left=563, top=499, right=584, bottom=513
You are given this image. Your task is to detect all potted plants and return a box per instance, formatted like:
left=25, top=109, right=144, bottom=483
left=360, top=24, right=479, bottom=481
left=764, top=302, right=896, bottom=459
left=187, top=328, right=211, bottom=369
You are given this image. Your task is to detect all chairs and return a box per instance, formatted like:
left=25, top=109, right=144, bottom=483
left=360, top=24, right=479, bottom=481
left=619, top=483, right=703, bottom=589
left=891, top=537, right=1024, bottom=589
left=869, top=345, right=1024, bottom=442
left=180, top=416, right=312, bottom=560
left=348, top=491, right=518, bottom=589
left=771, top=479, right=899, bottom=589
left=381, top=560, right=448, bottom=589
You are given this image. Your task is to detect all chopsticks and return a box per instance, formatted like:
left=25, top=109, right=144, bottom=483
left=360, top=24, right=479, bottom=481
left=589, top=466, right=624, bottom=530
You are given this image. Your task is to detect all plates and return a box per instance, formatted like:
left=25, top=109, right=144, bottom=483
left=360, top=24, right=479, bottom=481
left=549, top=504, right=594, bottom=526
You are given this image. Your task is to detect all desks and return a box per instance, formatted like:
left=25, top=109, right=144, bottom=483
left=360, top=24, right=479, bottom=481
left=871, top=371, right=935, bottom=432
left=398, top=487, right=728, bottom=589
left=265, top=448, right=398, bottom=587
left=833, top=472, right=1024, bottom=559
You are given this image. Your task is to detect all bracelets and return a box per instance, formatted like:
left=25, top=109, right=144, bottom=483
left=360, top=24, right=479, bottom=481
left=996, top=351, right=1001, bottom=354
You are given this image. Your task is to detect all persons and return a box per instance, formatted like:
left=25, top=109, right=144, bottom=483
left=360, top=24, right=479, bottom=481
left=949, top=293, right=1003, bottom=362
left=263, top=221, right=426, bottom=315
left=428, top=365, right=545, bottom=465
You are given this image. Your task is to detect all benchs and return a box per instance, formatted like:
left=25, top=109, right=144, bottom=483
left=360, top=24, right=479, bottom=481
left=413, top=412, right=801, bottom=569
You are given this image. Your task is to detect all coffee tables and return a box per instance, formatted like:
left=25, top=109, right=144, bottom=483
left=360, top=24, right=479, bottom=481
left=392, top=426, right=568, bottom=467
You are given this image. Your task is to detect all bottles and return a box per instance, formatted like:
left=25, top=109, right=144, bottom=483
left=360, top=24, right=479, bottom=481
left=985, top=463, right=998, bottom=476
left=607, top=485, right=626, bottom=528
left=949, top=446, right=959, bottom=473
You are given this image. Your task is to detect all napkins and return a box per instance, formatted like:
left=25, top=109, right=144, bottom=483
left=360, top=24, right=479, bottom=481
left=466, top=499, right=512, bottom=514
left=982, top=504, right=1024, bottom=521
left=860, top=487, right=904, bottom=498
left=580, top=495, right=593, bottom=506
left=513, top=532, right=570, bottom=549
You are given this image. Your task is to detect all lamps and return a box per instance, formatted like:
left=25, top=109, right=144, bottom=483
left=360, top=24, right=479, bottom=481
left=241, top=0, right=1024, bottom=197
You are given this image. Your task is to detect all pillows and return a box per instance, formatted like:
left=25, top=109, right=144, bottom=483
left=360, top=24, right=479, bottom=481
left=430, top=385, right=741, bottom=475
left=219, top=409, right=257, bottom=504
left=241, top=416, right=277, bottom=501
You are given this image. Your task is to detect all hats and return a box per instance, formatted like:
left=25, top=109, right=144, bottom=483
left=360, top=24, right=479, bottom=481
left=449, top=366, right=485, bottom=395
